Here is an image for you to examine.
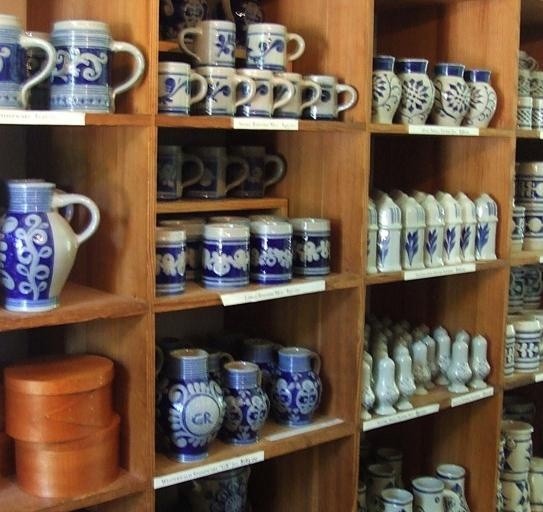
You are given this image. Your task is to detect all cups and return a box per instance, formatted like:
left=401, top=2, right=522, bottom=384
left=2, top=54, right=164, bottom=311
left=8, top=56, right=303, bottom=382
left=157, top=61, right=208, bottom=114
left=291, top=216, right=331, bottom=276
left=231, top=145, right=286, bottom=197
left=306, top=74, right=358, bottom=120
left=154, top=227, right=187, bottom=292
left=201, top=225, right=251, bottom=288
left=359, top=433, right=472, bottom=510
left=22, top=32, right=53, bottom=109
left=274, top=72, right=321, bottom=118
left=178, top=21, right=237, bottom=67
left=194, top=65, right=255, bottom=114
left=251, top=221, right=292, bottom=282
left=209, top=217, right=250, bottom=224
left=251, top=213, right=284, bottom=222
left=49, top=19, right=145, bottom=111
left=237, top=67, right=295, bottom=117
left=187, top=146, right=250, bottom=199
left=245, top=23, right=306, bottom=70
left=160, top=219, right=206, bottom=279
left=157, top=145, right=205, bottom=201
left=504, top=265, right=542, bottom=374
left=0, top=15, right=57, bottom=109
left=516, top=49, right=541, bottom=128
left=511, top=160, right=542, bottom=251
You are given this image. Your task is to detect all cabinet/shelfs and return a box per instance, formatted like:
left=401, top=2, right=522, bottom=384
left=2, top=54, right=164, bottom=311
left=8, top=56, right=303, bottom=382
left=1, top=0, right=153, bottom=512
left=355, top=1, right=525, bottom=511
left=156, top=1, right=373, bottom=512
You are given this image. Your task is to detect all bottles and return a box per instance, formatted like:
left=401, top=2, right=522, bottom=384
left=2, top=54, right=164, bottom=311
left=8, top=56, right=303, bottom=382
left=495, top=397, right=541, bottom=511
